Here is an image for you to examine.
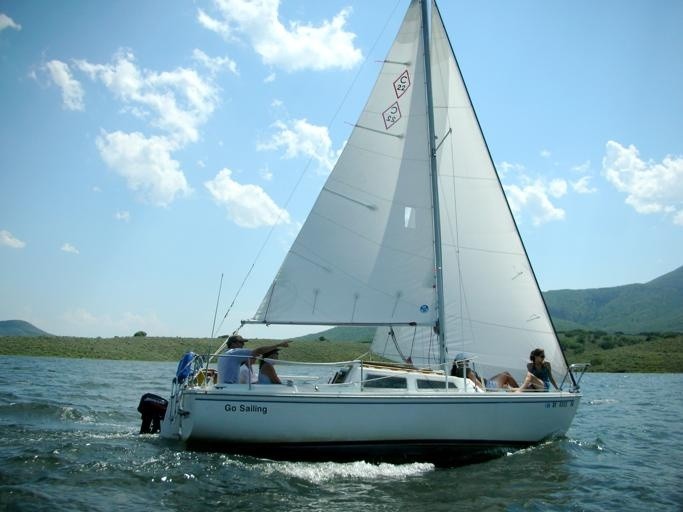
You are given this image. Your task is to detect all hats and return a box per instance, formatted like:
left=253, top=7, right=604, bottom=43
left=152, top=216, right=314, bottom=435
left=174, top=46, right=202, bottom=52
left=228, top=336, right=248, bottom=342
left=455, top=353, right=469, bottom=360
left=262, top=349, right=280, bottom=355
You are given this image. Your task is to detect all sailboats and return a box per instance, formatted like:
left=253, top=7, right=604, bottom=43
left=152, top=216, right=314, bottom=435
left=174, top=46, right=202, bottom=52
left=133, top=0, right=592, bottom=466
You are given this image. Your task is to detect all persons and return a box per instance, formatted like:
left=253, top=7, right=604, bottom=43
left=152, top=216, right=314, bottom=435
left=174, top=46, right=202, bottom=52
left=450, top=352, right=519, bottom=392
left=217, top=335, right=293, bottom=383
left=255, top=347, right=281, bottom=384
left=510, top=348, right=559, bottom=393
left=238, top=355, right=257, bottom=384
